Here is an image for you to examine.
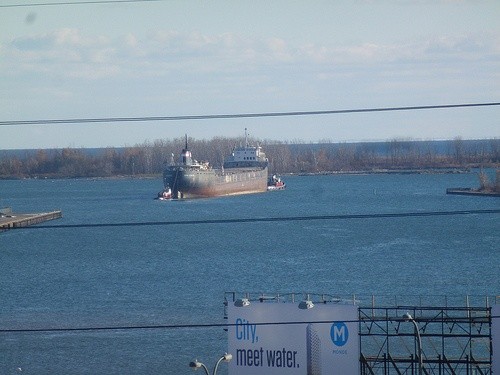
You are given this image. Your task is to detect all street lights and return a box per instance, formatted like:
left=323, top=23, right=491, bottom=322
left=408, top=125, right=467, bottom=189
left=403, top=312, right=423, bottom=375
left=188, top=352, right=233, bottom=375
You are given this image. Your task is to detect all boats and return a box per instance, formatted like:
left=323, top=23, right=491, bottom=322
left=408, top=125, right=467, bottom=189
left=267, top=172, right=288, bottom=192
left=154, top=127, right=269, bottom=201
